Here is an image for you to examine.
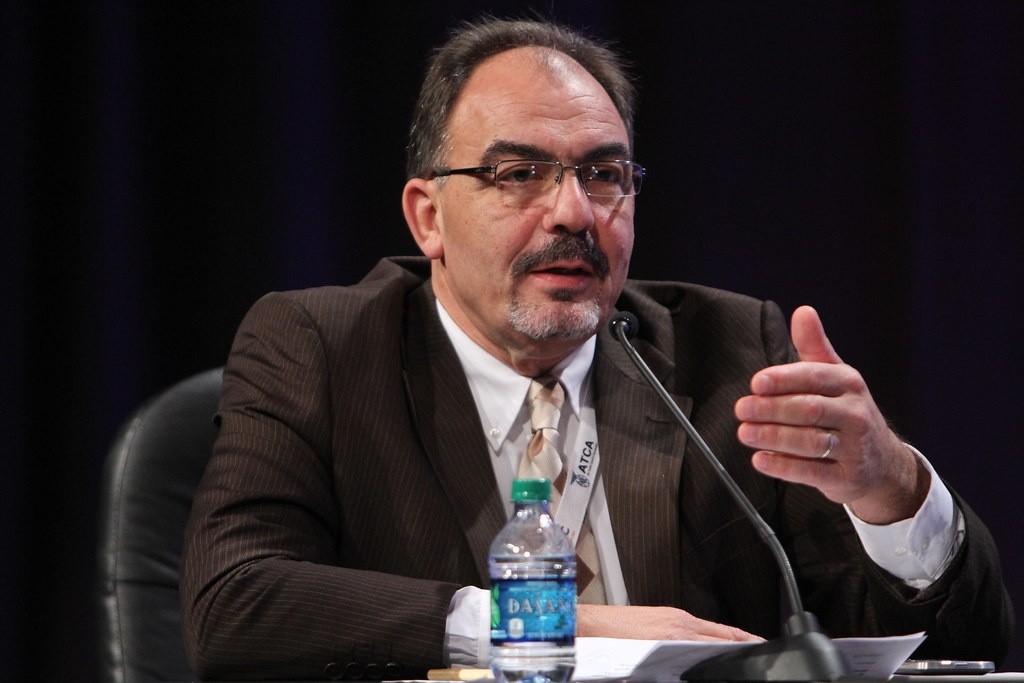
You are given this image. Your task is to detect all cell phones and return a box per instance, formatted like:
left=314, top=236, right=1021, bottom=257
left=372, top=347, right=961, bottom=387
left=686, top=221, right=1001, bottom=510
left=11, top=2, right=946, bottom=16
left=895, top=659, right=996, bottom=675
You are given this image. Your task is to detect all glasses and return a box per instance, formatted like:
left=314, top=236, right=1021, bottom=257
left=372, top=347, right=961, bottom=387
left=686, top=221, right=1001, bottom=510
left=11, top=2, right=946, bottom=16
left=418, top=159, right=645, bottom=197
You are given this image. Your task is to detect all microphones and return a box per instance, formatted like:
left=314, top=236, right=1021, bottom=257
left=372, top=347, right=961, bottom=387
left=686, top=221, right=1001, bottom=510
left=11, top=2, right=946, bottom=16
left=607, top=311, right=852, bottom=683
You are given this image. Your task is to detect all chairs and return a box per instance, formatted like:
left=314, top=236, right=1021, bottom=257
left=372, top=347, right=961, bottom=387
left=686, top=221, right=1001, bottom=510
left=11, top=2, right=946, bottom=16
left=86, top=368, right=225, bottom=683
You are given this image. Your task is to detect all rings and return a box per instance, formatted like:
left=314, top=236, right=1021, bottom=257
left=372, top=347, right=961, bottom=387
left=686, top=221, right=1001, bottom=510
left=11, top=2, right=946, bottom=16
left=820, top=430, right=833, bottom=458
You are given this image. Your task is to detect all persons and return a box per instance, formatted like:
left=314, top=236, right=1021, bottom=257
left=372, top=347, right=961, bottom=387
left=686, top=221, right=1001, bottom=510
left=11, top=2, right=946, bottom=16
left=181, top=17, right=1015, bottom=683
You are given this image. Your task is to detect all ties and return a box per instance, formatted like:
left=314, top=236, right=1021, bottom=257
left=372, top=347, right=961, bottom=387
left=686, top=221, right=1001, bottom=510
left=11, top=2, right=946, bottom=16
left=517, top=375, right=607, bottom=604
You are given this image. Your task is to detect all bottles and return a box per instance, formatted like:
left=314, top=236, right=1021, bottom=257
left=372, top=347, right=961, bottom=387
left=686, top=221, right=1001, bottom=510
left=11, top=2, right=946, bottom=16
left=489, top=478, right=577, bottom=683
